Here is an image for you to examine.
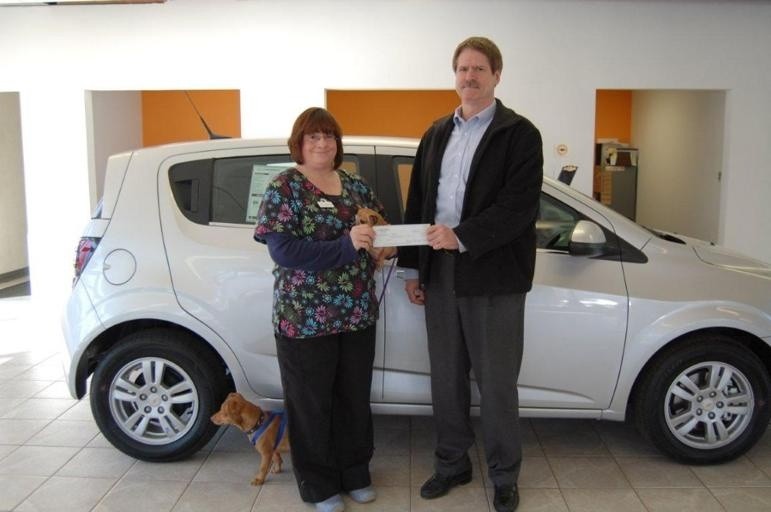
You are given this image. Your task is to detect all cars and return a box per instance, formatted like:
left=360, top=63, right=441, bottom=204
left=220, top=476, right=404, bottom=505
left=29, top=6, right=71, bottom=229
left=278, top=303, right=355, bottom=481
left=52, top=89, right=768, bottom=466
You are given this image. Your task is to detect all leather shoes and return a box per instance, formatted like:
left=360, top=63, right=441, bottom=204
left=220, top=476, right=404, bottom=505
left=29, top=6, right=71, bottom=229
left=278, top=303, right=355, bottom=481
left=494, top=483, right=519, bottom=511
left=420, top=472, right=472, bottom=499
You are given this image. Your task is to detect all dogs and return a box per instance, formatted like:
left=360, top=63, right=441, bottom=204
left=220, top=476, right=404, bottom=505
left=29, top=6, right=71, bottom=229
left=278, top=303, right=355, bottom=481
left=208, top=391, right=294, bottom=487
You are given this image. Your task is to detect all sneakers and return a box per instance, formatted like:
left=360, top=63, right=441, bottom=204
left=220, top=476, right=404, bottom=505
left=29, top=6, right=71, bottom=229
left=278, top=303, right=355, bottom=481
left=349, top=485, right=376, bottom=504
left=316, top=495, right=344, bottom=512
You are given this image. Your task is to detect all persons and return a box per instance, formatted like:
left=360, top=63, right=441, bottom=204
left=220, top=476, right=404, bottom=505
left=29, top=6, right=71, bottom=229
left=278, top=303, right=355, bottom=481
left=253, top=107, right=398, bottom=511
left=398, top=36, right=544, bottom=512
